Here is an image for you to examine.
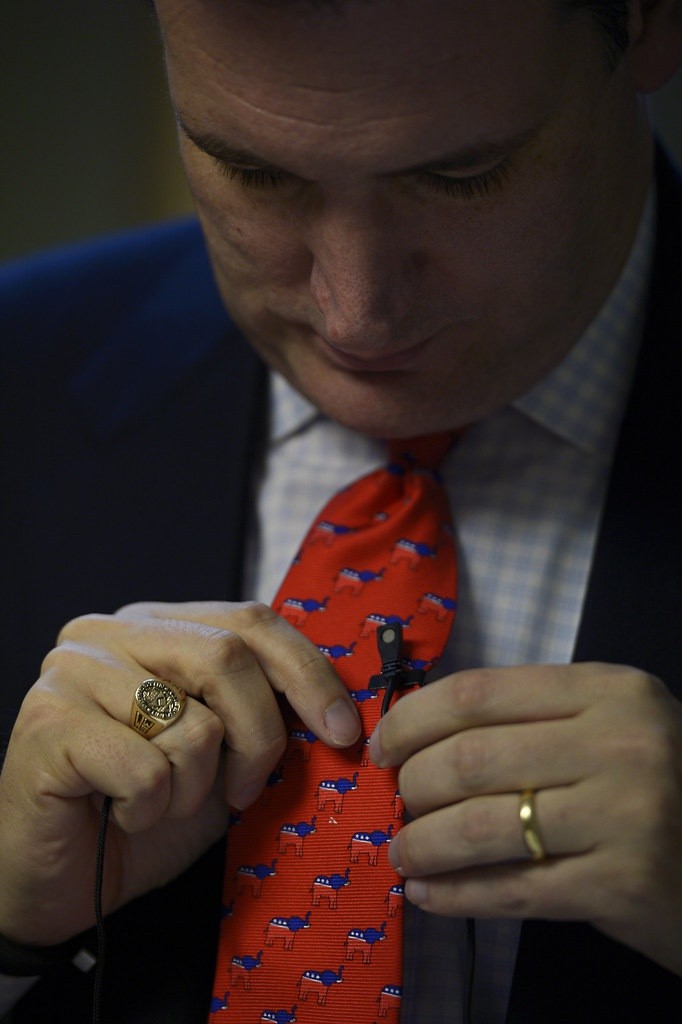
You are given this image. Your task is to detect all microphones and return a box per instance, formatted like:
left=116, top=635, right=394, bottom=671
left=377, top=619, right=402, bottom=681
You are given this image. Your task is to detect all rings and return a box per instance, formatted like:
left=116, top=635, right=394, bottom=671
left=517, top=791, right=550, bottom=862
left=129, top=678, right=186, bottom=738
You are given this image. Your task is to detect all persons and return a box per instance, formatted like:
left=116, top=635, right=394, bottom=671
left=0, top=0, right=681, bottom=1024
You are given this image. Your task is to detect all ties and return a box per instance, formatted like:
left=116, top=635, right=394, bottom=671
left=208, top=431, right=462, bottom=1023
